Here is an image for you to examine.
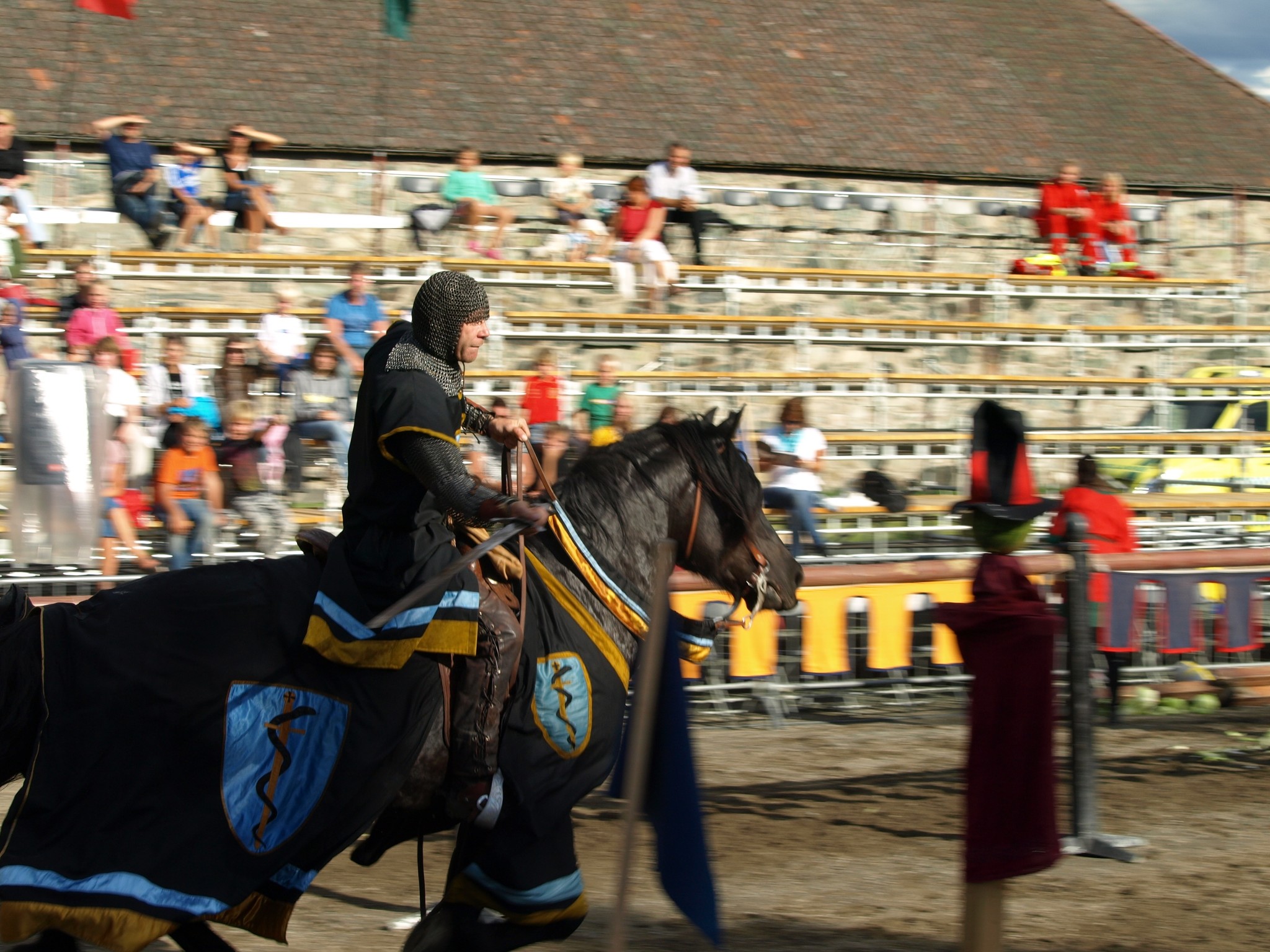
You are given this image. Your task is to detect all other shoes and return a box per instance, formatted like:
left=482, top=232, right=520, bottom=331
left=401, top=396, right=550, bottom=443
left=466, top=240, right=481, bottom=253
left=484, top=248, right=502, bottom=260
left=443, top=778, right=490, bottom=820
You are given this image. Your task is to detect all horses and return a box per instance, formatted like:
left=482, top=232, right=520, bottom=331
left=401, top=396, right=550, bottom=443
left=0, top=403, right=804, bottom=952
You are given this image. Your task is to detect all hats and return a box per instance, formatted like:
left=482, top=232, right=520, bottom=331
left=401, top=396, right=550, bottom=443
left=781, top=401, right=803, bottom=422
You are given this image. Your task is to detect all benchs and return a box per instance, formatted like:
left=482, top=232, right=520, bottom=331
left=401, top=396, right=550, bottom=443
left=0, top=175, right=1270, bottom=709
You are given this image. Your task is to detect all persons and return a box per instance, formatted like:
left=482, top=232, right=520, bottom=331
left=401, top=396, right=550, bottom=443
left=448, top=349, right=684, bottom=498
left=0, top=108, right=297, bottom=253
left=1034, top=158, right=1145, bottom=275
left=757, top=398, right=832, bottom=558
left=344, top=273, right=548, bottom=833
left=1, top=257, right=392, bottom=590
left=1047, top=452, right=1144, bottom=719
left=440, top=141, right=751, bottom=314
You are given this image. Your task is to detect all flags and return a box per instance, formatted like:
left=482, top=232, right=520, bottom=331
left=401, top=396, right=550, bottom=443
left=69, top=0, right=136, bottom=21
left=385, top=0, right=412, bottom=40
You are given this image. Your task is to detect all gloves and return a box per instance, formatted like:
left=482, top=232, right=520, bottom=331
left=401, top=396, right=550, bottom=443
left=507, top=501, right=550, bottom=536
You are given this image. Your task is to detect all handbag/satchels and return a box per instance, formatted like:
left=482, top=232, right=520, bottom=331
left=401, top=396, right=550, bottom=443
left=862, top=470, right=906, bottom=511
